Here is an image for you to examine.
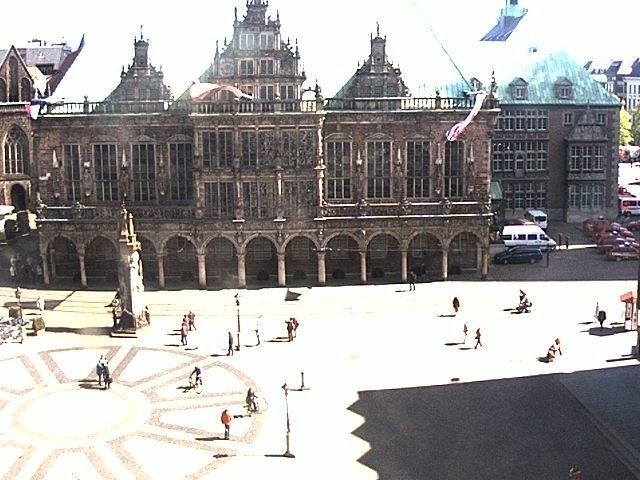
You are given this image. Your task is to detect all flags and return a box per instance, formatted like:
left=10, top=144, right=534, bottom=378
left=27, top=95, right=62, bottom=120
left=189, top=83, right=252, bottom=102
left=444, top=93, right=487, bottom=141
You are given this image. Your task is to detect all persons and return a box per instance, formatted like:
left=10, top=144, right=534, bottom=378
left=558, top=234, right=562, bottom=250
left=453, top=297, right=459, bottom=312
left=474, top=328, right=482, bottom=349
left=284, top=317, right=299, bottom=342
left=96, top=354, right=112, bottom=390
left=517, top=288, right=531, bottom=312
left=547, top=337, right=562, bottom=362
left=408, top=272, right=416, bottom=291
left=595, top=302, right=599, bottom=316
left=246, top=389, right=257, bottom=411
left=114, top=288, right=120, bottom=299
left=190, top=366, right=202, bottom=386
left=228, top=332, right=233, bottom=355
left=565, top=236, right=569, bottom=249
left=221, top=409, right=232, bottom=440
left=254, top=329, right=260, bottom=345
left=463, top=323, right=468, bottom=336
left=180, top=310, right=196, bottom=346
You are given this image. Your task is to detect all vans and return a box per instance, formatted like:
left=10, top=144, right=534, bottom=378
left=501, top=225, right=557, bottom=252
left=616, top=195, right=640, bottom=217
left=526, top=210, right=548, bottom=230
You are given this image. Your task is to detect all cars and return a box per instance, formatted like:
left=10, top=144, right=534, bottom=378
left=494, top=245, right=544, bottom=264
left=583, top=218, right=640, bottom=261
left=506, top=218, right=536, bottom=225
